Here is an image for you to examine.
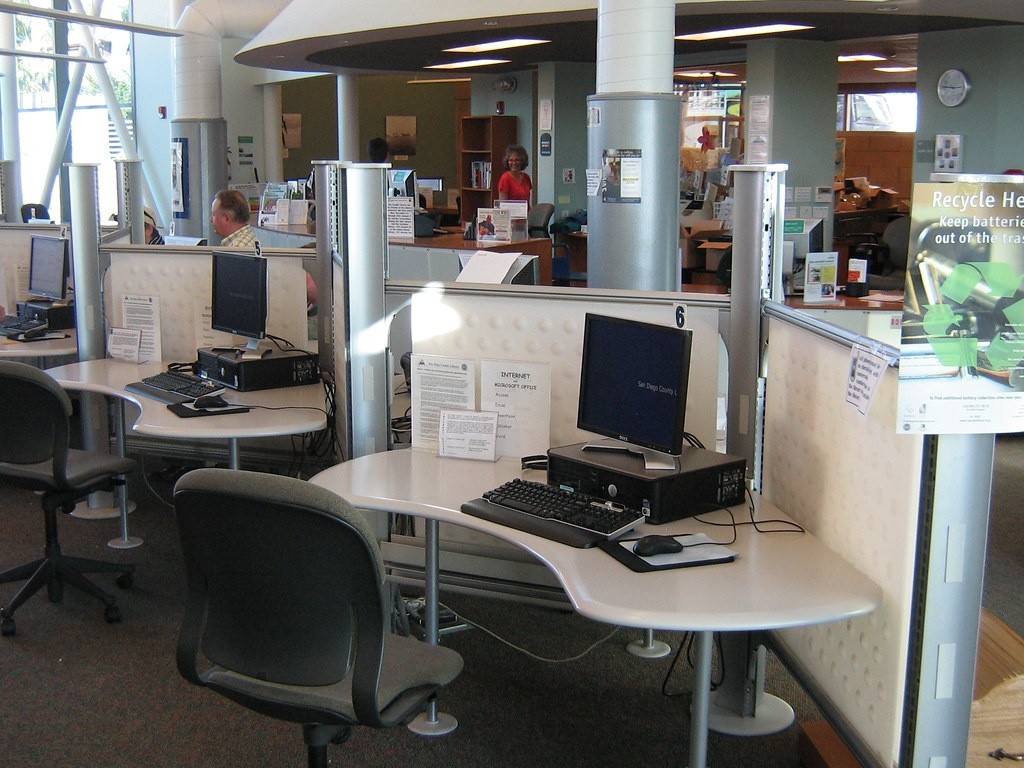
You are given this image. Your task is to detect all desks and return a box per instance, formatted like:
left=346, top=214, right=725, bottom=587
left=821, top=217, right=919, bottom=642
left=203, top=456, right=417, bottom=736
left=247, top=220, right=552, bottom=285
left=425, top=208, right=460, bottom=227
left=44, top=355, right=334, bottom=553
left=0, top=328, right=78, bottom=369
left=305, top=444, right=885, bottom=768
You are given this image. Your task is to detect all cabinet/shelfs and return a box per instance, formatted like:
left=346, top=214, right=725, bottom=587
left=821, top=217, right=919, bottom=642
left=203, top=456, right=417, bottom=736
left=459, top=114, right=517, bottom=227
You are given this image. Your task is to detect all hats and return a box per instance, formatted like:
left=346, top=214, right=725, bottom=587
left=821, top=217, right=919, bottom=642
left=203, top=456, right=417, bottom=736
left=144, top=206, right=164, bottom=228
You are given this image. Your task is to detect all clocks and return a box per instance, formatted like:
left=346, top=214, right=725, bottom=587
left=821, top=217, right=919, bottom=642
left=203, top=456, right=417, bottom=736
left=936, top=68, right=972, bottom=108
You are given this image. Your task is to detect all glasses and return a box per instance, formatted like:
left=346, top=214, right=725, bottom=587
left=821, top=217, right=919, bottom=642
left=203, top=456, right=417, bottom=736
left=508, top=159, right=521, bottom=164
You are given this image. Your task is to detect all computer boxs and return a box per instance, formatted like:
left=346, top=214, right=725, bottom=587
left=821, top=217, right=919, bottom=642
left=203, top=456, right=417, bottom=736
left=197, top=342, right=321, bottom=392
left=16, top=299, right=76, bottom=330
left=547, top=439, right=746, bottom=525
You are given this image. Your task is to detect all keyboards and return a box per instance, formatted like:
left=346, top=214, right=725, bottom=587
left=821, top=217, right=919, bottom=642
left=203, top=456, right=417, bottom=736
left=0, top=316, right=49, bottom=336
left=461, top=477, right=645, bottom=550
left=124, top=371, right=226, bottom=405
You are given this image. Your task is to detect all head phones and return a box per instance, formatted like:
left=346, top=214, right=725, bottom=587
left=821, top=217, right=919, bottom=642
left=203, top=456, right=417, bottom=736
left=167, top=360, right=198, bottom=374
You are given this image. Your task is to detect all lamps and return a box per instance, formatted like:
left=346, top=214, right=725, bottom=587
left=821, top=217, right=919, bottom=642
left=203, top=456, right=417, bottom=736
left=493, top=75, right=517, bottom=95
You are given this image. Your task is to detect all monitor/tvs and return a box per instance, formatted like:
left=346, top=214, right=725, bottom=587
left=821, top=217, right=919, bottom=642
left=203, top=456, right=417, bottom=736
left=211, top=250, right=273, bottom=359
left=576, top=312, right=693, bottom=471
left=28, top=234, right=74, bottom=306
left=417, top=176, right=445, bottom=191
left=388, top=169, right=419, bottom=215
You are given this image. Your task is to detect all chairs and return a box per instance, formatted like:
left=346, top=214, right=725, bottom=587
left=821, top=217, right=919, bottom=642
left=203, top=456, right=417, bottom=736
left=526, top=202, right=555, bottom=238
left=551, top=243, right=571, bottom=286
left=0, top=358, right=139, bottom=638
left=170, top=467, right=466, bottom=768
left=846, top=218, right=911, bottom=291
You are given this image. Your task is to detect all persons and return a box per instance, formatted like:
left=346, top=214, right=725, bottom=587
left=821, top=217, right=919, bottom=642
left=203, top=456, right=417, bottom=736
left=480, top=215, right=495, bottom=235
left=825, top=284, right=834, bottom=294
left=498, top=143, right=532, bottom=213
left=564, top=170, right=573, bottom=182
left=609, top=161, right=620, bottom=185
left=143, top=205, right=165, bottom=245
left=209, top=189, right=262, bottom=248
left=360, top=137, right=390, bottom=163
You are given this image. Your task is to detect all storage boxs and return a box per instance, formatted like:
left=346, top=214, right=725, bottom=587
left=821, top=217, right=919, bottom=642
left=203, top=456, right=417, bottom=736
left=680, top=220, right=724, bottom=268
left=713, top=203, right=721, bottom=220
left=876, top=188, right=900, bottom=208
left=692, top=272, right=717, bottom=285
left=697, top=239, right=732, bottom=271
left=703, top=183, right=728, bottom=201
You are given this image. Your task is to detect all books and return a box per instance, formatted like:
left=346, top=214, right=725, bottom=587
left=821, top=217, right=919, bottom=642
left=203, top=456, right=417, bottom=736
left=471, top=162, right=492, bottom=190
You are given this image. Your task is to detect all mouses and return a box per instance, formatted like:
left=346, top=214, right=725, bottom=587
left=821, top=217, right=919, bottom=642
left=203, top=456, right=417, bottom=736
left=633, top=535, right=683, bottom=556
left=24, top=330, right=46, bottom=338
left=193, top=396, right=228, bottom=408
